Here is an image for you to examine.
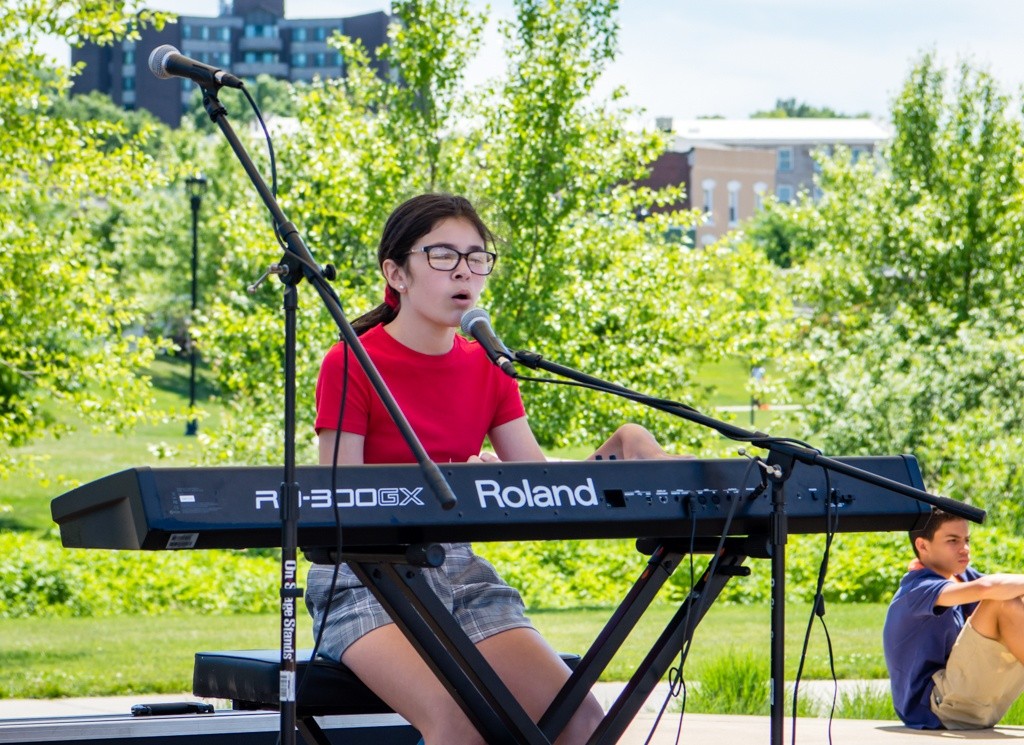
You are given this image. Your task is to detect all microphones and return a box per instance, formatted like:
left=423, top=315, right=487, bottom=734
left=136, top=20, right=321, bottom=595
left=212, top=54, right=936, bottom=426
left=461, top=308, right=517, bottom=378
left=149, top=44, right=245, bottom=90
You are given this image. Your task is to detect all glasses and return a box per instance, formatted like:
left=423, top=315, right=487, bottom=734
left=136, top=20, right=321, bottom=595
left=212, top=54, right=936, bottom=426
left=404, top=243, right=497, bottom=276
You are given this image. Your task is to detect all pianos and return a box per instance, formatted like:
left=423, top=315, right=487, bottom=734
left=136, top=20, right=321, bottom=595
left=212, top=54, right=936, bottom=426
left=50, top=454, right=932, bottom=745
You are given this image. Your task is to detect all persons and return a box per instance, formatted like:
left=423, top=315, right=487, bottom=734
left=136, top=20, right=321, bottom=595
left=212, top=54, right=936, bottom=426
left=884, top=508, right=1024, bottom=731
left=305, top=194, right=697, bottom=745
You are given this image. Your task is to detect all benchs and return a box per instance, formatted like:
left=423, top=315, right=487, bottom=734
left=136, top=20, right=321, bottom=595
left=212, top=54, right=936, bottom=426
left=192, top=647, right=582, bottom=745
